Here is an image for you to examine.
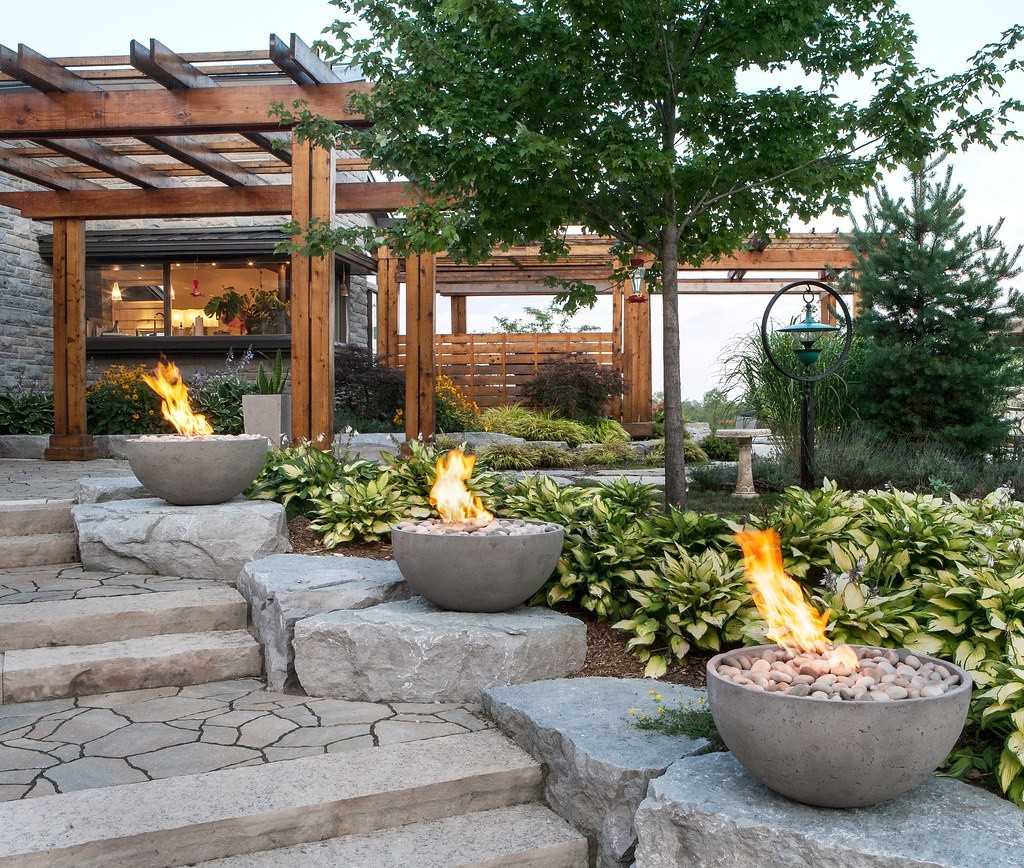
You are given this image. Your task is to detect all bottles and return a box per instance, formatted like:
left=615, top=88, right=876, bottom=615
left=112, top=320, right=120, bottom=333
left=191, top=323, right=195, bottom=336
left=195, top=316, right=204, bottom=336
left=179, top=322, right=183, bottom=329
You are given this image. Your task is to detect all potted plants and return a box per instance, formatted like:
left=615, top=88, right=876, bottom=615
left=242, top=349, right=290, bottom=450
left=204, top=285, right=286, bottom=335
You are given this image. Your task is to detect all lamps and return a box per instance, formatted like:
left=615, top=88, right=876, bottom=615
left=110, top=267, right=122, bottom=301
left=777, top=307, right=840, bottom=373
left=190, top=262, right=201, bottom=297
left=625, top=257, right=648, bottom=305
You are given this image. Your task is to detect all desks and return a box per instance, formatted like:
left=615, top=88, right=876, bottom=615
left=714, top=428, right=772, bottom=499
